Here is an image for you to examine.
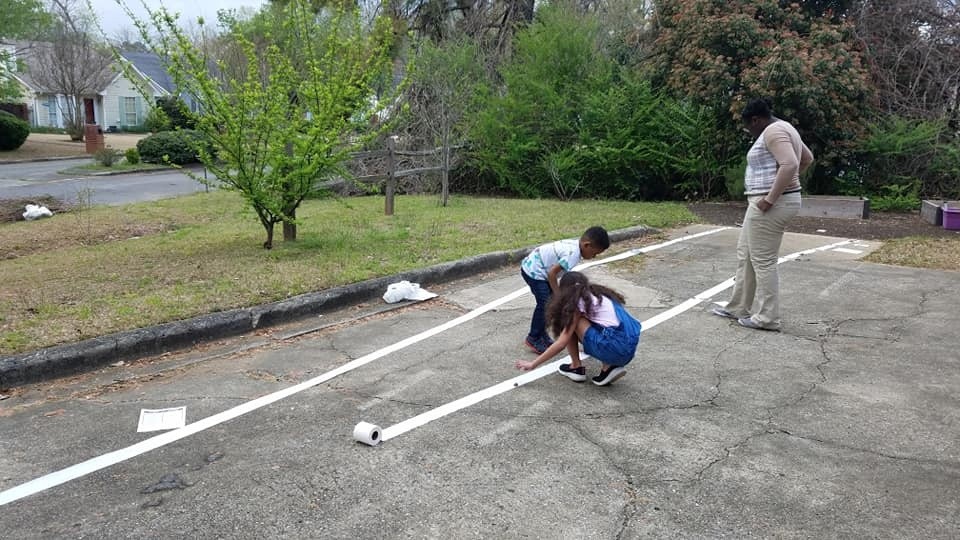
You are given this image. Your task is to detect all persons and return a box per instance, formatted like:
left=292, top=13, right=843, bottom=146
left=519, top=227, right=611, bottom=357
left=712, top=99, right=814, bottom=331
left=515, top=272, right=642, bottom=386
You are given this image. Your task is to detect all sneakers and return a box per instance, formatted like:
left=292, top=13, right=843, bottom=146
left=711, top=308, right=751, bottom=320
left=526, top=332, right=555, bottom=355
left=591, top=364, right=626, bottom=386
left=559, top=364, right=587, bottom=381
left=737, top=317, right=762, bottom=329
